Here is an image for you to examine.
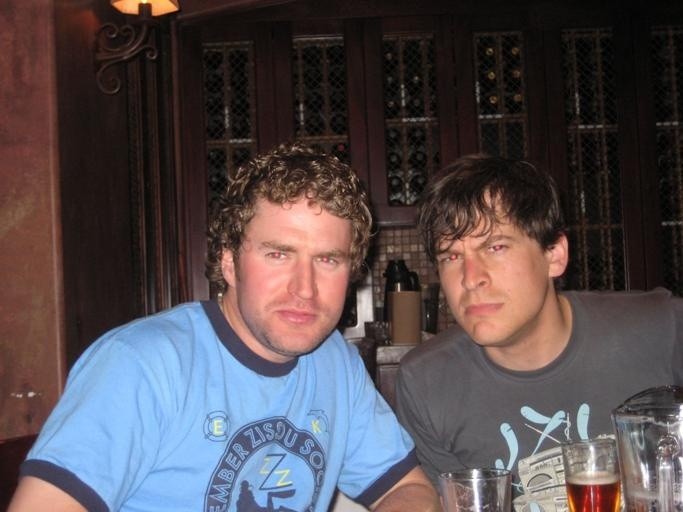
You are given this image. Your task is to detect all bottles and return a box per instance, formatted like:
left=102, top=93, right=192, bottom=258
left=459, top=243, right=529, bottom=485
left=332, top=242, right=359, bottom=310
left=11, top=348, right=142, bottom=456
left=381, top=259, right=422, bottom=347
left=203, top=42, right=523, bottom=209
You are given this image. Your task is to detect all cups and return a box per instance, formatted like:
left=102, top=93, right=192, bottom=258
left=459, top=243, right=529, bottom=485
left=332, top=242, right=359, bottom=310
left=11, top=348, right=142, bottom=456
left=364, top=321, right=392, bottom=346
left=560, top=438, right=622, bottom=512
left=609, top=383, right=683, bottom=512
left=436, top=468, right=513, bottom=512
left=419, top=282, right=441, bottom=335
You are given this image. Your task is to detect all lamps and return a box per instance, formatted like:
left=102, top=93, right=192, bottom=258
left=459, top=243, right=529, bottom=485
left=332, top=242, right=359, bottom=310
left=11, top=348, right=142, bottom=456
left=92, top=0, right=182, bottom=97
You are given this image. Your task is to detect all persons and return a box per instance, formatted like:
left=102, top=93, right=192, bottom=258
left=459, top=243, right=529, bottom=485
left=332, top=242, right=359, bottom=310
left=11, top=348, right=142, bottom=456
left=393, top=153, right=683, bottom=512
left=6, top=140, right=445, bottom=512
